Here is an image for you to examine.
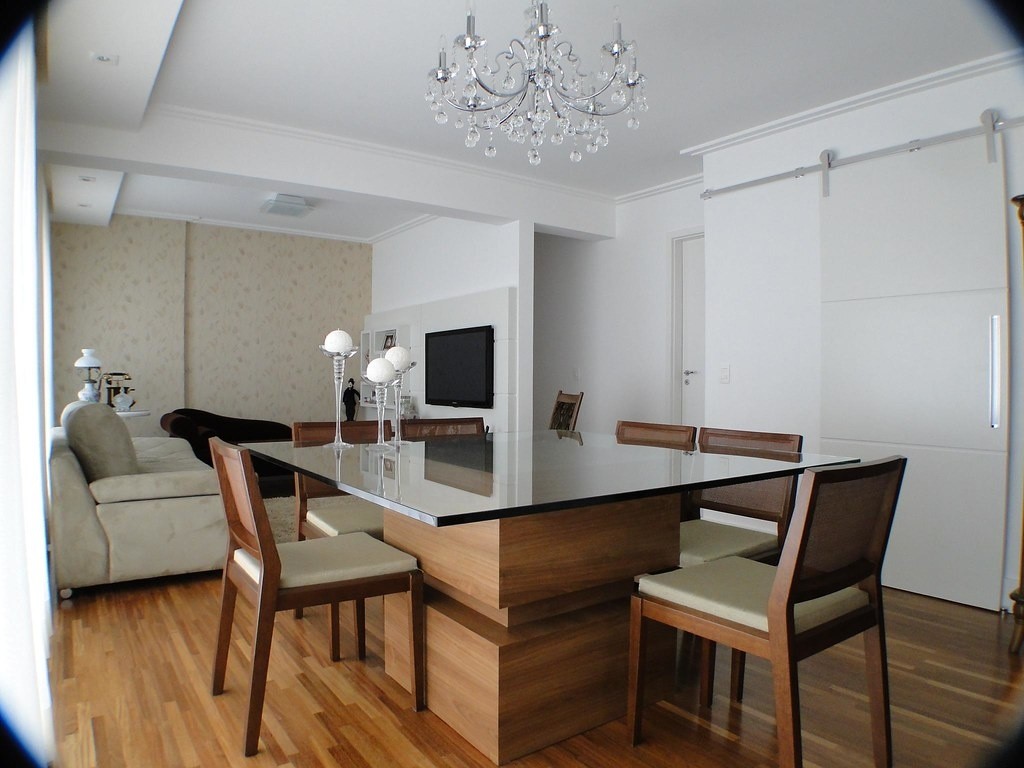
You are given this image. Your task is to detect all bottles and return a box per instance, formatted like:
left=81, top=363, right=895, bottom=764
left=405, top=404, right=415, bottom=419
left=112, top=386, right=133, bottom=411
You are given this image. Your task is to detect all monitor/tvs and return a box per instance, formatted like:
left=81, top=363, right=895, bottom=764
left=425, top=325, right=494, bottom=409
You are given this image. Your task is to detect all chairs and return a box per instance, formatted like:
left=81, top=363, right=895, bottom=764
left=548, top=390, right=583, bottom=431
left=614, top=421, right=697, bottom=522
left=208, top=436, right=426, bottom=757
left=293, top=417, right=488, bottom=660
left=679, top=426, right=805, bottom=703
left=627, top=455, right=907, bottom=768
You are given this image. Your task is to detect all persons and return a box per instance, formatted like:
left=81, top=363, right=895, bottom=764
left=343, top=378, right=360, bottom=422
left=385, top=336, right=393, bottom=349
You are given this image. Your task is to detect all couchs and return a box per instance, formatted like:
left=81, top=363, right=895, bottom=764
left=49, top=401, right=229, bottom=597
left=160, top=408, right=295, bottom=498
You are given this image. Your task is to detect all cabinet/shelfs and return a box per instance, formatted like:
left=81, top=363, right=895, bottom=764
left=361, top=328, right=411, bottom=409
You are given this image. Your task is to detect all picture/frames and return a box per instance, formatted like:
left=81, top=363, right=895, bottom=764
left=381, top=333, right=396, bottom=351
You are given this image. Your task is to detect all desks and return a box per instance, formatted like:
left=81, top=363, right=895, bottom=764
left=240, top=430, right=862, bottom=766
left=116, top=410, right=151, bottom=433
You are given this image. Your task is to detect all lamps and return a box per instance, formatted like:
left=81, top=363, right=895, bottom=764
left=74, top=348, right=102, bottom=402
left=425, top=0, right=650, bottom=167
left=261, top=195, right=317, bottom=220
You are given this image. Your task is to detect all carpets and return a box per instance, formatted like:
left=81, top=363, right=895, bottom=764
left=263, top=496, right=296, bottom=545
